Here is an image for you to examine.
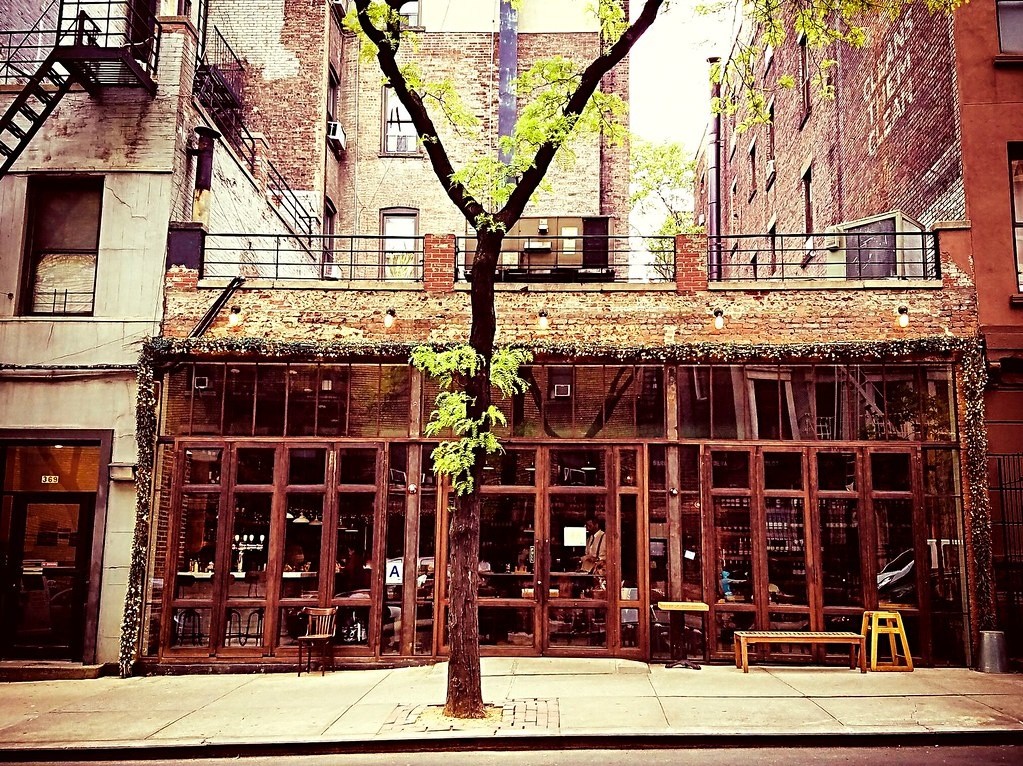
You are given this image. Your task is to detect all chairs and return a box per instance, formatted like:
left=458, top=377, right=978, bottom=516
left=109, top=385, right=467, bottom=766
left=297, top=608, right=337, bottom=677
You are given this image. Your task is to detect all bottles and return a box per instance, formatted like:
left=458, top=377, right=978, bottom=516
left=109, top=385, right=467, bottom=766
left=189, top=559, right=202, bottom=572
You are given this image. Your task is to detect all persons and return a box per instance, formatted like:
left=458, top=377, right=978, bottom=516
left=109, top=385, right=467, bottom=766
left=339, top=540, right=365, bottom=592
left=572, top=516, right=607, bottom=599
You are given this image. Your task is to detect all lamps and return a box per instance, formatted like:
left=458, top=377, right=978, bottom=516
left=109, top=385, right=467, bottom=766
left=319, top=368, right=335, bottom=396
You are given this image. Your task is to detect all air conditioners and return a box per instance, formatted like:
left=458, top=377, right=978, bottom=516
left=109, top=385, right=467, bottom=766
left=327, top=121, right=347, bottom=150
left=825, top=208, right=926, bottom=281
left=323, top=262, right=343, bottom=280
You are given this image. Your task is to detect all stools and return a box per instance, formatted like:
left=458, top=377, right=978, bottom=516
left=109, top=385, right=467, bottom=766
left=658, top=602, right=709, bottom=670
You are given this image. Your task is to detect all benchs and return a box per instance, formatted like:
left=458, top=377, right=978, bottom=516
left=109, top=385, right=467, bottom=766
left=734, top=631, right=867, bottom=674
